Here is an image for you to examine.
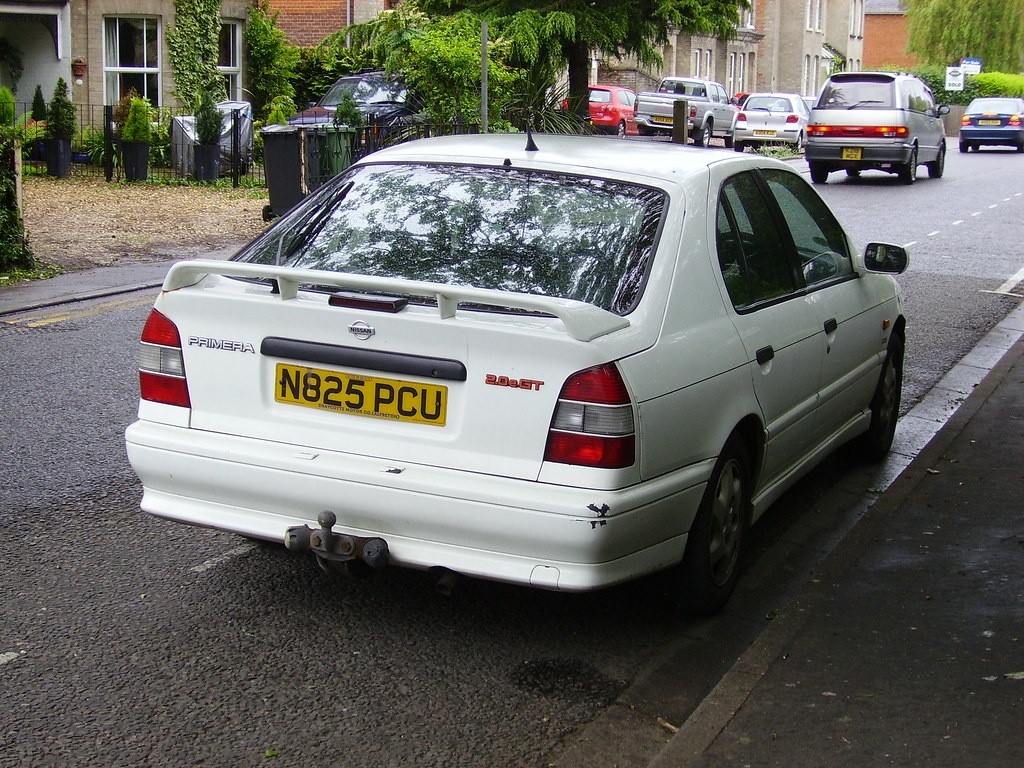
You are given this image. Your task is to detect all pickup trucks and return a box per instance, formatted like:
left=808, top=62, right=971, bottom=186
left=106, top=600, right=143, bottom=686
left=632, top=76, right=740, bottom=149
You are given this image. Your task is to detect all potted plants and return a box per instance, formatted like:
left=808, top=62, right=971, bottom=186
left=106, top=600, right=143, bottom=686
left=193, top=85, right=220, bottom=182
left=42, top=78, right=78, bottom=178
left=71, top=56, right=87, bottom=76
left=120, top=97, right=154, bottom=183
left=72, top=125, right=96, bottom=163
left=30, top=85, right=49, bottom=161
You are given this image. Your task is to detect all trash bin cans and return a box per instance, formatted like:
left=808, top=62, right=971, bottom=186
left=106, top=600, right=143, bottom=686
left=322, top=126, right=356, bottom=177
left=258, top=123, right=324, bottom=221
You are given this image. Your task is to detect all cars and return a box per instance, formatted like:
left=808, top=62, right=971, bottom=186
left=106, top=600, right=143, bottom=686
left=959, top=97, right=1024, bottom=154
left=560, top=83, right=640, bottom=137
left=122, top=132, right=911, bottom=628
left=730, top=92, right=818, bottom=152
left=262, top=66, right=430, bottom=164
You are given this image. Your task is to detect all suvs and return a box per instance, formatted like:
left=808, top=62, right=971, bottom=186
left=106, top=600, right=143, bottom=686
left=804, top=71, right=951, bottom=185
